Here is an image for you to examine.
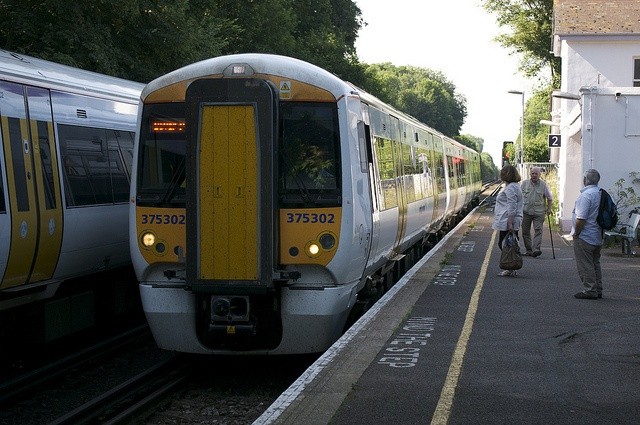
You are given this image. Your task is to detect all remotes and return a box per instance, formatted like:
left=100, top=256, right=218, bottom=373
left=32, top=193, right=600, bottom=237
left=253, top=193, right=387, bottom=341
left=615, top=90, right=622, bottom=97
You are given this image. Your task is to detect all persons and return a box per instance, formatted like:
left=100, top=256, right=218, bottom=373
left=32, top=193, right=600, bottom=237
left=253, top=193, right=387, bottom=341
left=492, top=165, right=524, bottom=277
left=570, top=168, right=608, bottom=300
left=519, top=166, right=552, bottom=257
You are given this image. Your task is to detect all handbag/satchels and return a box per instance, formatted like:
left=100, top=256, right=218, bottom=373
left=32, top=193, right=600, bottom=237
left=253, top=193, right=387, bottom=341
left=501, top=238, right=520, bottom=250
left=499, top=246, right=522, bottom=270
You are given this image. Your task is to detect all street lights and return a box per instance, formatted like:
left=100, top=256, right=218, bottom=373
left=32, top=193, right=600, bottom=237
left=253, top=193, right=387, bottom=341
left=507, top=90, right=524, bottom=181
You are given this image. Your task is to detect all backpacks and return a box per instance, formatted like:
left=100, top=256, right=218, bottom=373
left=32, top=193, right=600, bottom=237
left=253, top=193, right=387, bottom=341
left=597, top=188, right=618, bottom=230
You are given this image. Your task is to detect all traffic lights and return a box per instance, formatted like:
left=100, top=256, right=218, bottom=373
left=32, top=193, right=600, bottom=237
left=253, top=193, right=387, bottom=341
left=501, top=140, right=515, bottom=165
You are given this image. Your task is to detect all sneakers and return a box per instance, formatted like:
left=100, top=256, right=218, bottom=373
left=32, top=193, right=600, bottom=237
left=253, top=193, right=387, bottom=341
left=497, top=270, right=517, bottom=277
left=531, top=250, right=542, bottom=257
left=574, top=292, right=602, bottom=299
left=521, top=250, right=532, bottom=256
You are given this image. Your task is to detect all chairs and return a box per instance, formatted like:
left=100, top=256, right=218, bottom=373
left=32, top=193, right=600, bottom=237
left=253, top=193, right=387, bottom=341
left=605, top=212, right=639, bottom=253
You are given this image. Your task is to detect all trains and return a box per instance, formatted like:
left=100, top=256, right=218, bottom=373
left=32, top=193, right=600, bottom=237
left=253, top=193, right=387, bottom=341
left=129, top=53, right=482, bottom=355
left=0, top=48, right=147, bottom=312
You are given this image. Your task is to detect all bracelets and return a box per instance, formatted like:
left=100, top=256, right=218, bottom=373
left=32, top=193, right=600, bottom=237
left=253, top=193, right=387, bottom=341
left=574, top=231, right=581, bottom=236
